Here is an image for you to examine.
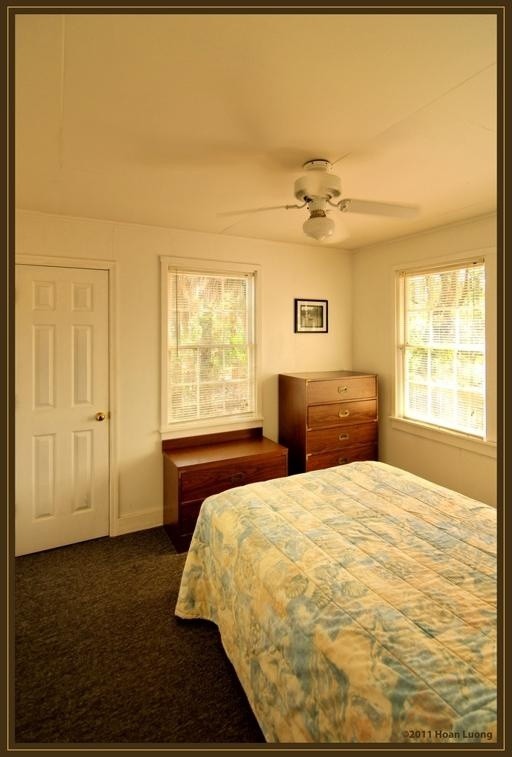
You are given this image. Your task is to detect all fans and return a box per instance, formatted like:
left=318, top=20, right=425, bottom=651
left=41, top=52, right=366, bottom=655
left=208, top=160, right=422, bottom=240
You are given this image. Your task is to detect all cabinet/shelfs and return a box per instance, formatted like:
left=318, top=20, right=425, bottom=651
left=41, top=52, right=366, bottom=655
left=280, top=372, right=380, bottom=471
left=161, top=434, right=289, bottom=555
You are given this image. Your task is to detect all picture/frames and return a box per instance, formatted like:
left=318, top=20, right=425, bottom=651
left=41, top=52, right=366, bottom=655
left=292, top=298, right=329, bottom=333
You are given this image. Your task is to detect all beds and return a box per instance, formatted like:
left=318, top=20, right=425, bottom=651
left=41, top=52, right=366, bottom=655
left=174, top=458, right=496, bottom=741
